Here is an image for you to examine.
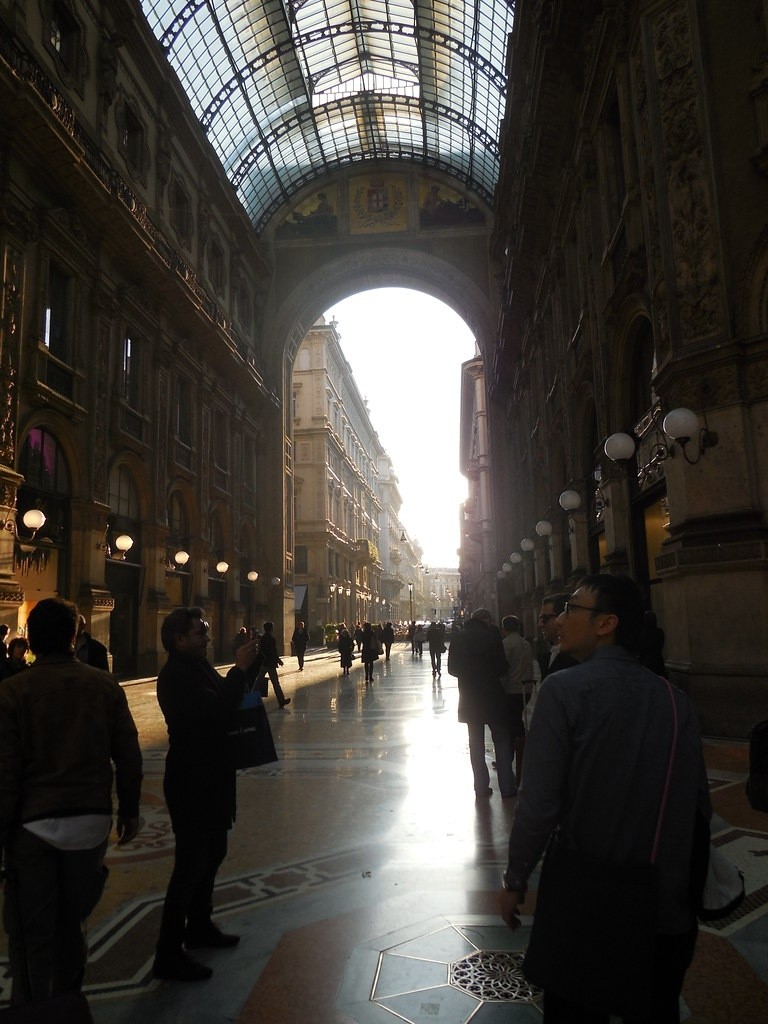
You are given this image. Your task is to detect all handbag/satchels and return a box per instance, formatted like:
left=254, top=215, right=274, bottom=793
left=261, top=678, right=268, bottom=697
left=377, top=648, right=384, bottom=655
left=226, top=690, right=278, bottom=770
left=523, top=831, right=658, bottom=1020
left=441, top=645, right=447, bottom=653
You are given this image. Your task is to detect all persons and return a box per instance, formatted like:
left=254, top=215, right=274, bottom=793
left=499, top=575, right=712, bottom=1024
left=337, top=621, right=394, bottom=681
left=292, top=621, right=310, bottom=671
left=410, top=621, right=444, bottom=675
left=151, top=606, right=259, bottom=982
left=452, top=593, right=667, bottom=798
left=0, top=613, right=109, bottom=682
left=0, top=598, right=142, bottom=1009
left=232, top=623, right=290, bottom=707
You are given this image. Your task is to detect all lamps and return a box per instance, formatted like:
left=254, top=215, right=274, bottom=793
left=272, top=576, right=281, bottom=587
left=521, top=538, right=539, bottom=563
left=663, top=382, right=719, bottom=466
left=328, top=584, right=372, bottom=601
left=96, top=521, right=133, bottom=560
left=558, top=463, right=610, bottom=524
left=496, top=562, right=513, bottom=580
left=236, top=571, right=258, bottom=585
left=510, top=552, right=525, bottom=572
left=0, top=495, right=45, bottom=543
left=161, top=546, right=189, bottom=575
left=204, top=558, right=228, bottom=579
left=536, top=520, right=574, bottom=550
left=605, top=404, right=674, bottom=492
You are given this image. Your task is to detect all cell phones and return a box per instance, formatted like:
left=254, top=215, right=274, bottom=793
left=252, top=629, right=258, bottom=640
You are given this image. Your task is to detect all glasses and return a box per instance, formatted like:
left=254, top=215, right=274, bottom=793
left=565, top=601, right=610, bottom=616
left=539, top=614, right=557, bottom=623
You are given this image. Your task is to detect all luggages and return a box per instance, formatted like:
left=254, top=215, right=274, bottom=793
left=516, top=680, right=537, bottom=788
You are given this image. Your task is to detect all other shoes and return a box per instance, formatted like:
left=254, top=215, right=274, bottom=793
left=184, top=921, right=240, bottom=947
left=280, top=698, right=291, bottom=707
left=153, top=951, right=212, bottom=981
left=501, top=790, right=516, bottom=799
left=370, top=678, right=374, bottom=681
left=365, top=678, right=369, bottom=680
left=476, top=788, right=493, bottom=797
